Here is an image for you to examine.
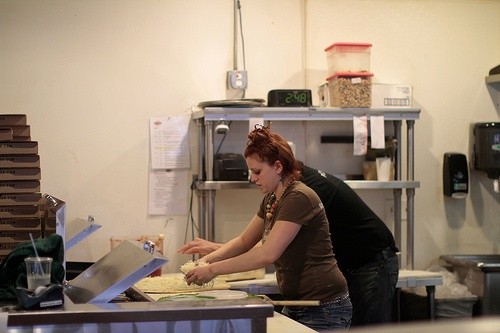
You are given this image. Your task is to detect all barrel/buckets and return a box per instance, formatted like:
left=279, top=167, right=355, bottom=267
left=109, top=234, right=163, bottom=278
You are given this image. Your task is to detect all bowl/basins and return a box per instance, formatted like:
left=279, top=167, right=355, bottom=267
left=268, top=89, right=312, bottom=107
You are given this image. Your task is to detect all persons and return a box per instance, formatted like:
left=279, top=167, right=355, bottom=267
left=186, top=127, right=353, bottom=333
left=179, top=160, right=402, bottom=326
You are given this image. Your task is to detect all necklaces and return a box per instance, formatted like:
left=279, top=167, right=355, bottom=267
left=263, top=175, right=296, bottom=243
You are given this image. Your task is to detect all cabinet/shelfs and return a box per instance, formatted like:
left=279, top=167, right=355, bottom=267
left=187, top=104, right=444, bottom=324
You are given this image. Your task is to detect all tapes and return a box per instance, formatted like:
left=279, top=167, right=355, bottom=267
left=35, top=285, right=47, bottom=295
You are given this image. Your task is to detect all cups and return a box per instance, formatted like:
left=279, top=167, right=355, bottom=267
left=25, top=257, right=53, bottom=289
left=362, top=150, right=376, bottom=181
left=376, top=150, right=396, bottom=181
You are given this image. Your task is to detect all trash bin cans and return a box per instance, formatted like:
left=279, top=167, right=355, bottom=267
left=396, top=286, right=478, bottom=321
left=439, top=254, right=500, bottom=317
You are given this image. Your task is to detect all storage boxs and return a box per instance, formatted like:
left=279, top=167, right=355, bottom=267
left=325, top=72, right=375, bottom=107
left=317, top=83, right=329, bottom=105
left=0, top=112, right=42, bottom=263
left=325, top=41, right=374, bottom=75
left=372, top=83, right=413, bottom=108
